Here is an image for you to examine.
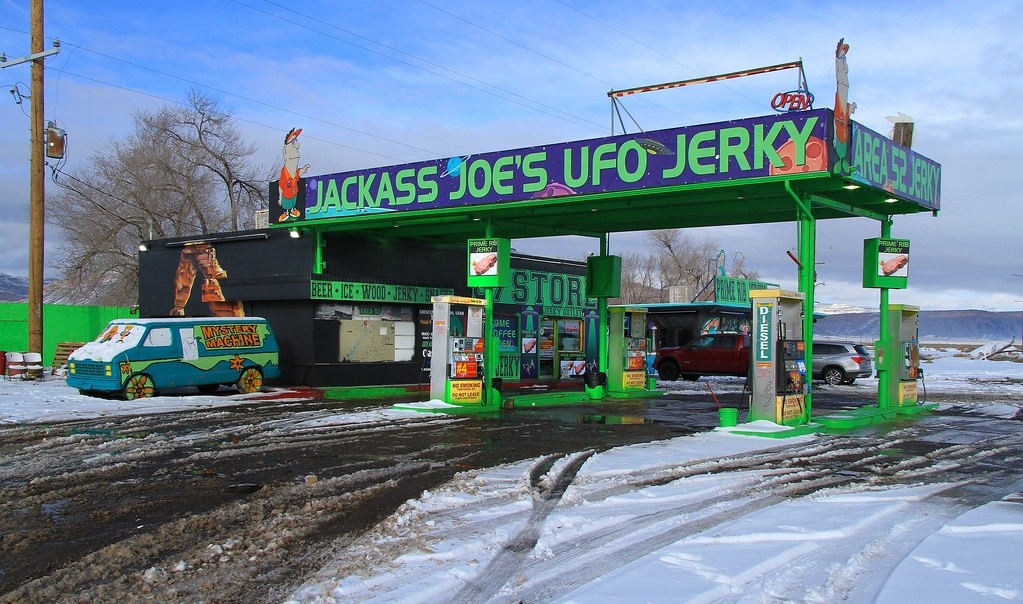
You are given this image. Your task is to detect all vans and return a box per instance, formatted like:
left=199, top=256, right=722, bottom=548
left=65, top=316, right=281, bottom=401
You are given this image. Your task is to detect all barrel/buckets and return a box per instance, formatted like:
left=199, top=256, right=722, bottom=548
left=718, top=408, right=738, bottom=427
left=646, top=378, right=656, bottom=390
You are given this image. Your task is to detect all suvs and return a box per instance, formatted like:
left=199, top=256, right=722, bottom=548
left=812, top=340, right=873, bottom=386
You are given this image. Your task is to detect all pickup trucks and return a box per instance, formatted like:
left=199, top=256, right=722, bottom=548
left=652, top=330, right=751, bottom=383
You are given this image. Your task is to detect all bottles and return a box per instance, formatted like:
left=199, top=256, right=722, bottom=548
left=912, top=336, right=916, bottom=341
